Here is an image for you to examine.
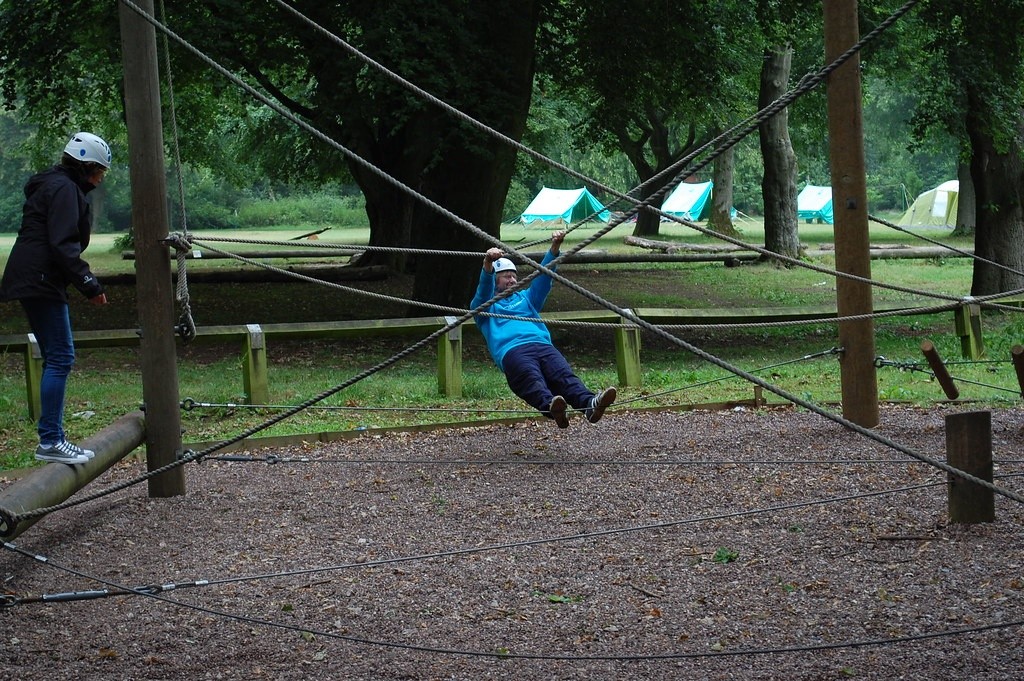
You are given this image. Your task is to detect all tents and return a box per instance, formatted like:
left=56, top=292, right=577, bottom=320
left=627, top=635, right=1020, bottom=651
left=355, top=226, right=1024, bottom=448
left=795, top=184, right=838, bottom=224
left=897, top=179, right=963, bottom=230
left=632, top=178, right=759, bottom=225
left=500, top=185, right=619, bottom=233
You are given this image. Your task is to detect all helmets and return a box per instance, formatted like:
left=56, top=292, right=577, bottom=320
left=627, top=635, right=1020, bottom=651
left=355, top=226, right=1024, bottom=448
left=63, top=132, right=111, bottom=170
left=492, top=257, right=517, bottom=272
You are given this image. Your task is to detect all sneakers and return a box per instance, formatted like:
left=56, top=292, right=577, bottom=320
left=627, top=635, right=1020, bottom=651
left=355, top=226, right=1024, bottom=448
left=34, top=439, right=95, bottom=464
left=549, top=396, right=569, bottom=429
left=586, top=388, right=616, bottom=423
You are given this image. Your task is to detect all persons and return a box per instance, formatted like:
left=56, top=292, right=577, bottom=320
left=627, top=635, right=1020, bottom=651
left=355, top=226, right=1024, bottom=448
left=470, top=231, right=617, bottom=429
left=0, top=132, right=112, bottom=464
